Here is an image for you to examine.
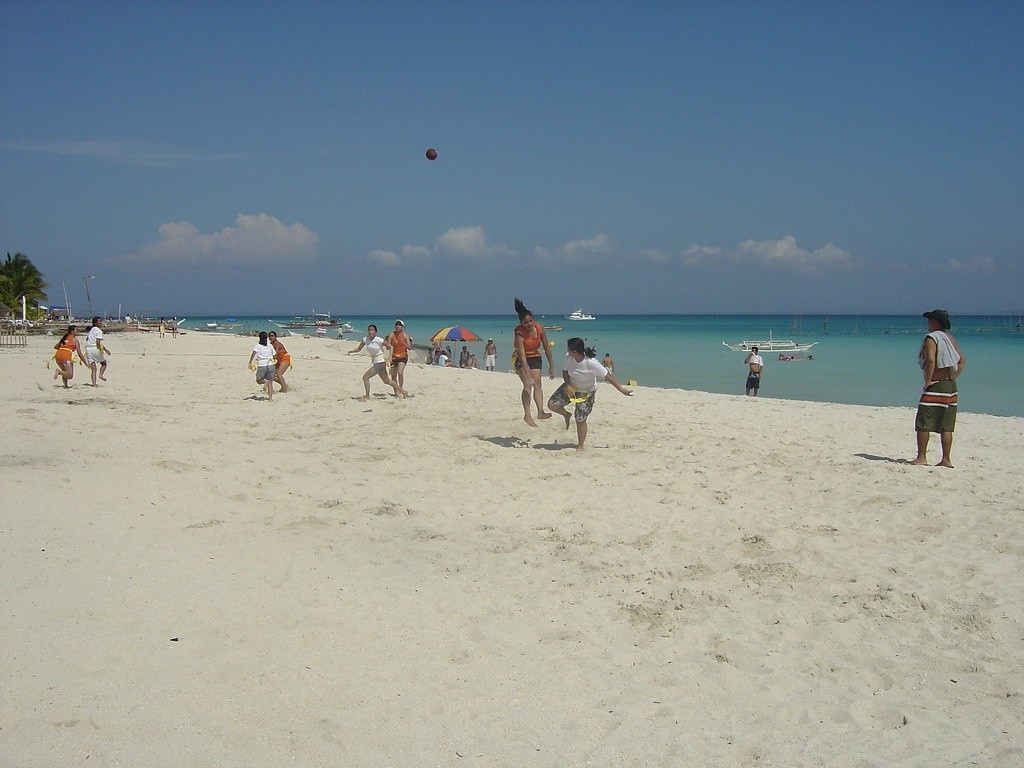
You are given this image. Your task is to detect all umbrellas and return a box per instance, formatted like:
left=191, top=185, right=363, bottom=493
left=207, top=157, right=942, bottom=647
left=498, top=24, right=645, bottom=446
left=431, top=326, right=484, bottom=367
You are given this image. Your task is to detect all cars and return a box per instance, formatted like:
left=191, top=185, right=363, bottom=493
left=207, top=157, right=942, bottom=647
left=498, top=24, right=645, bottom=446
left=0, top=317, right=35, bottom=326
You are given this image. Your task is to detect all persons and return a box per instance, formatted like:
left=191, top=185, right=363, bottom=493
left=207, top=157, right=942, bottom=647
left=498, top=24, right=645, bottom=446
left=159, top=316, right=168, bottom=338
left=511, top=298, right=555, bottom=427
left=54, top=316, right=111, bottom=389
left=483, top=338, right=497, bottom=371
left=743, top=347, right=763, bottom=397
left=386, top=320, right=412, bottom=397
left=459, top=345, right=480, bottom=371
left=779, top=353, right=814, bottom=361
left=602, top=352, right=615, bottom=383
left=171, top=318, right=179, bottom=339
left=248, top=331, right=290, bottom=403
left=337, top=326, right=343, bottom=340
left=425, top=340, right=452, bottom=367
left=125, top=313, right=137, bottom=324
left=547, top=338, right=633, bottom=451
left=911, top=310, right=965, bottom=468
left=348, top=325, right=409, bottom=400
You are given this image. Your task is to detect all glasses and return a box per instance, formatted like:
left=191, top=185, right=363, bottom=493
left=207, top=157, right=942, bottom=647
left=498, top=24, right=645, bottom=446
left=395, top=323, right=402, bottom=326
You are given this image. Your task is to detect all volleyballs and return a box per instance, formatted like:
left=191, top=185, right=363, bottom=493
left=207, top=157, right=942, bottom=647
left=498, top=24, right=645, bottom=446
left=426, top=148, right=438, bottom=160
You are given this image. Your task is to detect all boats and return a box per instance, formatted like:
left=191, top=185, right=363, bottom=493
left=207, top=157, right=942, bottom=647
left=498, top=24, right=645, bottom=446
left=567, top=308, right=595, bottom=321
left=268, top=314, right=352, bottom=327
left=544, top=324, right=563, bottom=331
left=722, top=340, right=820, bottom=352
left=129, top=319, right=186, bottom=333
left=194, top=323, right=244, bottom=331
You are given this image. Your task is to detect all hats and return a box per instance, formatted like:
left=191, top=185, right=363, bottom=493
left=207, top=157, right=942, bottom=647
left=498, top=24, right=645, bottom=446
left=488, top=338, right=493, bottom=341
left=394, top=319, right=404, bottom=326
left=923, top=310, right=952, bottom=330
